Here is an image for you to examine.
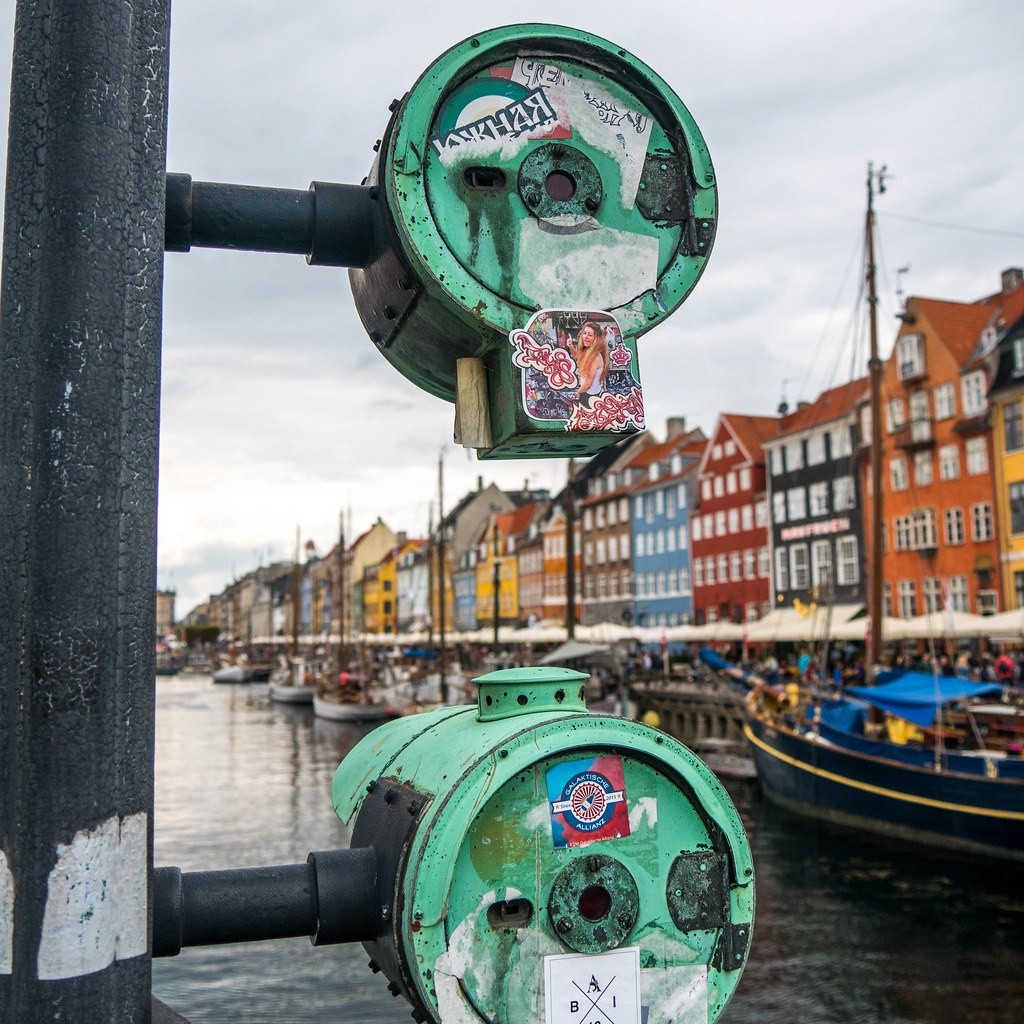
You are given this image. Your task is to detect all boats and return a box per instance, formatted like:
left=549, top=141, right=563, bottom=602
left=210, top=663, right=258, bottom=684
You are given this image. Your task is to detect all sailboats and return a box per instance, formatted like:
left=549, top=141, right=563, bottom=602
left=266, top=523, right=319, bottom=702
left=739, top=161, right=1024, bottom=868
left=381, top=457, right=470, bottom=717
left=312, top=505, right=383, bottom=720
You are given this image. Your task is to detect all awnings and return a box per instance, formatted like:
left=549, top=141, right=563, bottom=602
left=759, top=603, right=864, bottom=625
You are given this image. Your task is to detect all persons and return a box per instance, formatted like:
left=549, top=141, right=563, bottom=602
left=567, top=322, right=609, bottom=409
left=243, top=646, right=1022, bottom=705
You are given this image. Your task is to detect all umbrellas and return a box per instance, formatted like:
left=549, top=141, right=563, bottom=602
left=232, top=612, right=1023, bottom=645
